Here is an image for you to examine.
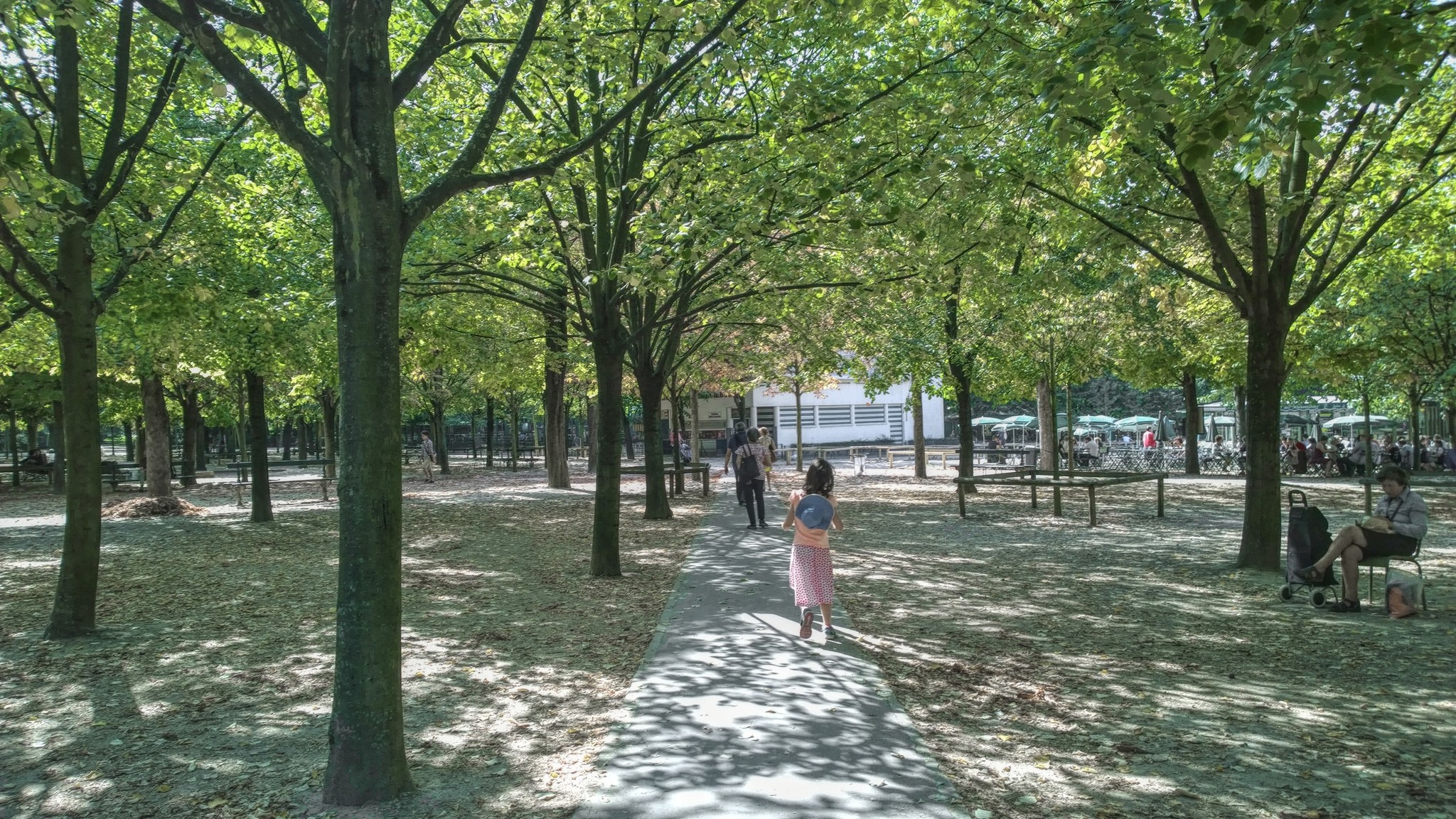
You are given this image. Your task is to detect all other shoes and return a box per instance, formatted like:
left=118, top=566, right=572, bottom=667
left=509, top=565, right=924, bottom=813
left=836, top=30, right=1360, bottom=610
left=1203, top=465, right=1446, bottom=478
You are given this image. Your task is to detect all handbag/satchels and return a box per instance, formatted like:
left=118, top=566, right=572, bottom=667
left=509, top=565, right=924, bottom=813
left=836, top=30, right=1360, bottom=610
left=770, top=449, right=778, bottom=463
left=1386, top=567, right=1428, bottom=619
left=739, top=443, right=760, bottom=482
left=429, top=454, right=434, bottom=462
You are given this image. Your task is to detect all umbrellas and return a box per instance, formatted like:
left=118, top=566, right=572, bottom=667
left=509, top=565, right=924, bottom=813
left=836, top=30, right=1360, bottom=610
left=1162, top=415, right=1175, bottom=447
left=1110, top=414, right=1159, bottom=456
left=1153, top=409, right=1169, bottom=450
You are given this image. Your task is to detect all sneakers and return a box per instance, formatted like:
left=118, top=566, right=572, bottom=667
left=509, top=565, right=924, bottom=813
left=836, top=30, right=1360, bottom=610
left=824, top=626, right=836, bottom=639
left=424, top=478, right=431, bottom=483
left=429, top=480, right=434, bottom=483
left=739, top=500, right=743, bottom=506
left=1329, top=598, right=1361, bottom=612
left=759, top=519, right=768, bottom=526
left=1292, top=565, right=1324, bottom=582
left=800, top=609, right=814, bottom=638
left=746, top=523, right=757, bottom=529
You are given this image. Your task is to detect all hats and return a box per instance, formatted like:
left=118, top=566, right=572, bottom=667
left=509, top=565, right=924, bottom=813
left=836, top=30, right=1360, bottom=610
left=795, top=494, right=835, bottom=530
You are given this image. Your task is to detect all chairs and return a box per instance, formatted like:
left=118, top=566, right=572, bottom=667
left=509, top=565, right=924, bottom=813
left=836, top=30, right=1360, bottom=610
left=1338, top=535, right=1428, bottom=613
left=1088, top=448, right=1103, bottom=473
left=1058, top=450, right=1073, bottom=472
left=1212, top=447, right=1403, bottom=475
left=1073, top=448, right=1090, bottom=472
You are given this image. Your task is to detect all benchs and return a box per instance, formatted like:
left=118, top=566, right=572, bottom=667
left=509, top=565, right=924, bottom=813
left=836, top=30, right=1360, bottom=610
left=401, top=444, right=589, bottom=471
left=105, top=459, right=199, bottom=493
left=225, top=454, right=339, bottom=506
left=949, top=445, right=1031, bottom=482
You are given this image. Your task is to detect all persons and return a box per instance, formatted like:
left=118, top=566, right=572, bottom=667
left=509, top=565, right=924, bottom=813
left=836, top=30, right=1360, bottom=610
left=780, top=457, right=843, bottom=639
left=969, top=410, right=1116, bottom=472
left=724, top=422, right=749, bottom=507
left=1175, top=436, right=1185, bottom=456
left=755, top=426, right=776, bottom=491
left=1127, top=433, right=1133, bottom=444
left=734, top=428, right=773, bottom=530
left=669, top=426, right=683, bottom=464
left=418, top=430, right=437, bottom=483
left=1292, top=465, right=1427, bottom=614
left=1122, top=433, right=1131, bottom=446
left=1142, top=425, right=1157, bottom=467
left=20, top=448, right=48, bottom=476
left=1201, top=411, right=1456, bottom=474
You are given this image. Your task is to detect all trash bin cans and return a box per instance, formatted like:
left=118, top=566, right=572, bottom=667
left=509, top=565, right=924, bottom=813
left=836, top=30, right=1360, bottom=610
left=1024, top=445, right=1040, bottom=470
